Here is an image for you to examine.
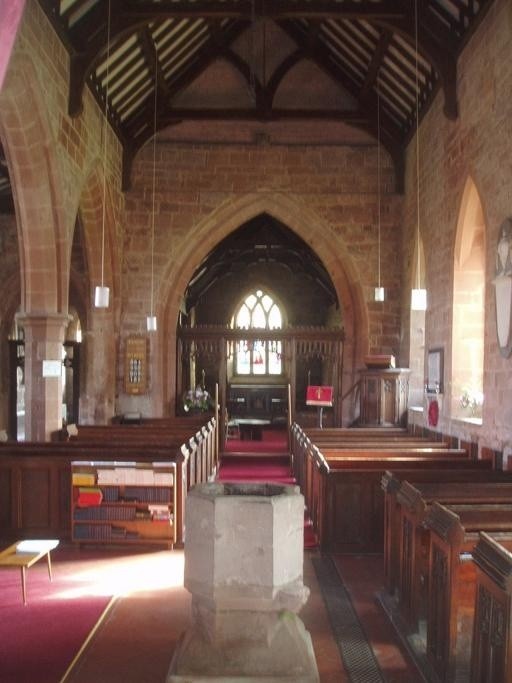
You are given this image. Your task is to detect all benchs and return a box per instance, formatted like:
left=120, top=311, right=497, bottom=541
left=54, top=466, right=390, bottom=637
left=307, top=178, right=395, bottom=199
left=0, top=417, right=218, bottom=550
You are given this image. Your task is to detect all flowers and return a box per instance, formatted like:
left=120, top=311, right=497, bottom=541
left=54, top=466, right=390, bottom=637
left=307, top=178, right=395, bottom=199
left=184, top=388, right=209, bottom=412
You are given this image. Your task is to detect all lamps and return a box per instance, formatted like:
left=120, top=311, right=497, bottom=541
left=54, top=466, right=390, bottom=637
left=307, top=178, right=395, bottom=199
left=63, top=318, right=83, bottom=367
left=7, top=311, right=26, bottom=369
left=375, top=69, right=385, bottom=303
left=147, top=42, right=157, bottom=333
left=94, top=0, right=110, bottom=310
left=411, top=2, right=427, bottom=312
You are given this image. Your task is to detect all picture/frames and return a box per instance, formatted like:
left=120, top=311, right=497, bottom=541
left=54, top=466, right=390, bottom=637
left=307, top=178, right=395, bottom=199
left=494, top=218, right=512, bottom=358
left=428, top=347, right=444, bottom=396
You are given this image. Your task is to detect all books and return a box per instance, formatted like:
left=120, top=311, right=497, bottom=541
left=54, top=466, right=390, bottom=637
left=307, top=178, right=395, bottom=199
left=72, top=465, right=172, bottom=540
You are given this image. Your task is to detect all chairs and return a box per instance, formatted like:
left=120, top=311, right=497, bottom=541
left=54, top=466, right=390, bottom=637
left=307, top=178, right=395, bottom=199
left=224, top=407, right=239, bottom=448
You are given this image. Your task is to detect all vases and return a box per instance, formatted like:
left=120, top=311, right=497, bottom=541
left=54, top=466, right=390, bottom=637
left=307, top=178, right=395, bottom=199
left=188, top=404, right=201, bottom=416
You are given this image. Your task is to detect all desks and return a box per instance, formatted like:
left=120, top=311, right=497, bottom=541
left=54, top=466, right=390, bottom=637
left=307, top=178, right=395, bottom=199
left=0, top=540, right=61, bottom=606
left=235, top=418, right=272, bottom=440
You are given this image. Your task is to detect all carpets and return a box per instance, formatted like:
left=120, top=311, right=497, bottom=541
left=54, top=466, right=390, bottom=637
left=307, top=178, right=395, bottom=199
left=1, top=568, right=119, bottom=682
left=217, top=457, right=319, bottom=547
left=226, top=427, right=288, bottom=454
